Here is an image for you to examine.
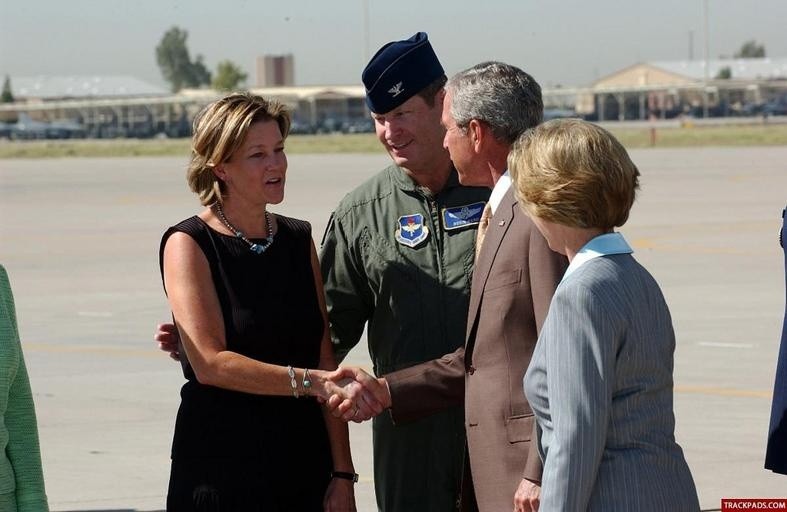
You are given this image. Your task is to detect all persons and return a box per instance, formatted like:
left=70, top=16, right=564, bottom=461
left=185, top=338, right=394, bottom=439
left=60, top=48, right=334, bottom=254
left=158, top=93, right=358, bottom=511
left=0, top=267, right=50, bottom=511
left=762, top=209, right=787, bottom=476
left=506, top=118, right=702, bottom=512
left=316, top=61, right=570, bottom=512
left=154, top=34, right=495, bottom=511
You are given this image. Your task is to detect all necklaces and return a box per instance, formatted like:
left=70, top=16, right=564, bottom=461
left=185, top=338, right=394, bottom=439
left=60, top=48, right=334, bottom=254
left=210, top=201, right=274, bottom=253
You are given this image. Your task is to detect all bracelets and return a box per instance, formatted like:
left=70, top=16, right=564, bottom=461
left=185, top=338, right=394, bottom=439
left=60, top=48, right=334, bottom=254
left=302, top=368, right=313, bottom=399
left=287, top=365, right=300, bottom=400
left=330, top=470, right=360, bottom=484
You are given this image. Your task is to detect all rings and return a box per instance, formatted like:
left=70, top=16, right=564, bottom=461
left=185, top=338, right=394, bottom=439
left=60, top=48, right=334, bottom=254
left=355, top=408, right=361, bottom=418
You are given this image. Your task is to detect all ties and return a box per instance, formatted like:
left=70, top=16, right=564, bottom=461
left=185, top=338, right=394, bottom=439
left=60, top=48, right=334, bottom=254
left=473, top=201, right=493, bottom=267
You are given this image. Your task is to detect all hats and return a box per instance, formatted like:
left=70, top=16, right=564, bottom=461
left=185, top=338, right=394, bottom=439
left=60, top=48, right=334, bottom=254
left=361, top=32, right=447, bottom=116
